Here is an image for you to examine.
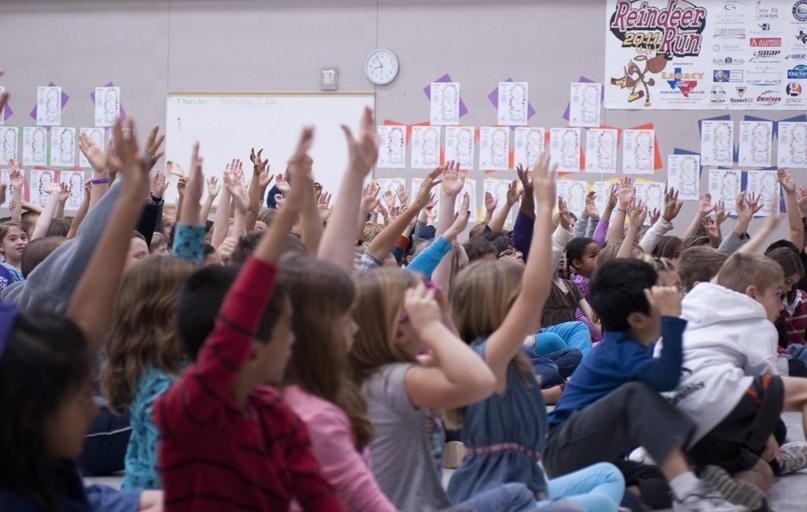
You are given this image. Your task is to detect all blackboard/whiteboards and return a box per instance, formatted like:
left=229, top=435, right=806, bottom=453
left=161, top=91, right=375, bottom=212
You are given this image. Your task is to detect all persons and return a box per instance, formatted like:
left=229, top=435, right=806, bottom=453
left=0, top=105, right=805, bottom=511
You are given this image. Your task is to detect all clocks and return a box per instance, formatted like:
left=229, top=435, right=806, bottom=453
left=364, top=48, right=398, bottom=87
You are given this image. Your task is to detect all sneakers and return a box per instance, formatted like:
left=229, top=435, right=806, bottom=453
left=699, top=464, right=770, bottom=512
left=670, top=479, right=751, bottom=512
left=778, top=441, right=807, bottom=474
